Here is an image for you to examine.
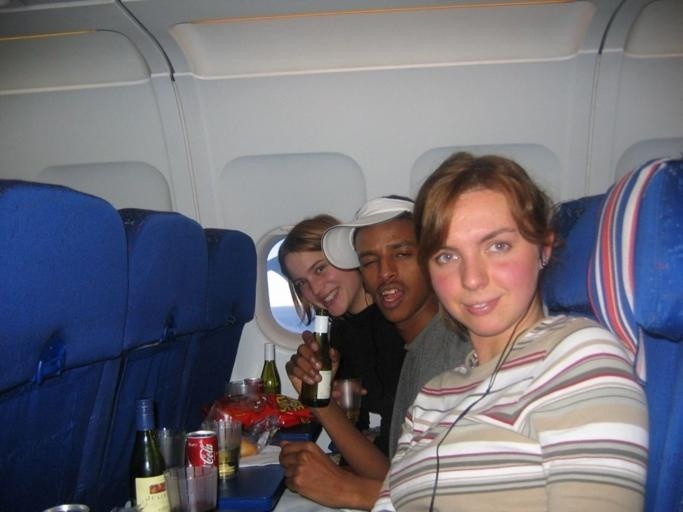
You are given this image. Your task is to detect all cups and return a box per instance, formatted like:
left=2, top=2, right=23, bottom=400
left=156, top=427, right=185, bottom=468
left=162, top=465, right=217, bottom=511
left=335, top=377, right=363, bottom=423
left=201, top=419, right=241, bottom=478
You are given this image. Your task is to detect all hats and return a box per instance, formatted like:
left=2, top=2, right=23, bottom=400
left=320, top=197, right=417, bottom=272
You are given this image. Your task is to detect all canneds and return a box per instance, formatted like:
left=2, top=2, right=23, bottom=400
left=185, top=430, right=218, bottom=504
left=230, top=378, right=263, bottom=396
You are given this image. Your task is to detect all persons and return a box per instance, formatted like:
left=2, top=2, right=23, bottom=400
left=275, top=213, right=408, bottom=463
left=364, top=151, right=652, bottom=512
left=264, top=192, right=479, bottom=512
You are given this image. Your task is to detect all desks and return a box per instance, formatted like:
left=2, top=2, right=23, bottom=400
left=109, top=400, right=325, bottom=510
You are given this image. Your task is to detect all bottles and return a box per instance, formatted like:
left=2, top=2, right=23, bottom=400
left=259, top=342, right=281, bottom=395
left=129, top=398, right=171, bottom=511
left=299, top=308, right=332, bottom=408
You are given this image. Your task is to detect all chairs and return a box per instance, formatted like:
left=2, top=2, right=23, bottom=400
left=541, top=156, right=682, bottom=510
left=0, top=180, right=257, bottom=511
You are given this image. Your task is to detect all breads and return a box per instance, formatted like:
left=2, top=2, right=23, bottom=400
left=240, top=437, right=258, bottom=458
